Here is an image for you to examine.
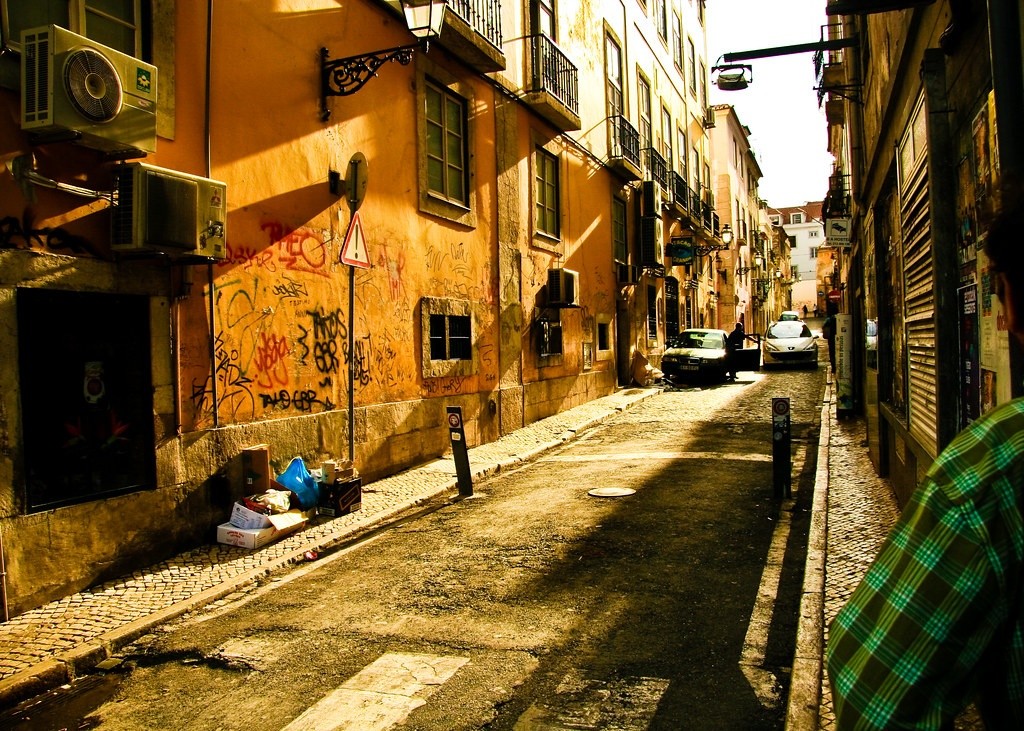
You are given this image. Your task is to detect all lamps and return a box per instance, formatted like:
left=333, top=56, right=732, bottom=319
left=319, top=0, right=448, bottom=123
left=694, top=224, right=733, bottom=257
left=770, top=267, right=781, bottom=281
left=737, top=252, right=763, bottom=275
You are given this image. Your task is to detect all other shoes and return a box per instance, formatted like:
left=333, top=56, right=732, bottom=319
left=734, top=377, right=739, bottom=379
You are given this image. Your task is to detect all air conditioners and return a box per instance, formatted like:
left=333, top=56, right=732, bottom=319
left=108, top=161, right=227, bottom=263
left=18, top=23, right=159, bottom=158
left=617, top=264, right=639, bottom=285
left=642, top=180, right=662, bottom=218
left=546, top=267, right=580, bottom=307
left=640, top=214, right=665, bottom=267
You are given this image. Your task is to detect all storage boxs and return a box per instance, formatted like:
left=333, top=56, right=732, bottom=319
left=217, top=512, right=307, bottom=550
left=230, top=488, right=309, bottom=530
left=241, top=444, right=272, bottom=495
left=297, top=505, right=318, bottom=523
left=317, top=476, right=363, bottom=517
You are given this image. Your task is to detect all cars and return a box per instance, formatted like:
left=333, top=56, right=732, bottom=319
left=660, top=328, right=761, bottom=383
left=760, top=321, right=820, bottom=371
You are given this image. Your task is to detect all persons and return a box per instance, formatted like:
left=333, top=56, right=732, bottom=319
left=824, top=187, right=1024, bottom=731
left=821, top=308, right=837, bottom=373
left=727, top=321, right=759, bottom=381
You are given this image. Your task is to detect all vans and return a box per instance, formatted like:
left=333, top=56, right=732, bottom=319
left=778, top=310, right=800, bottom=321
